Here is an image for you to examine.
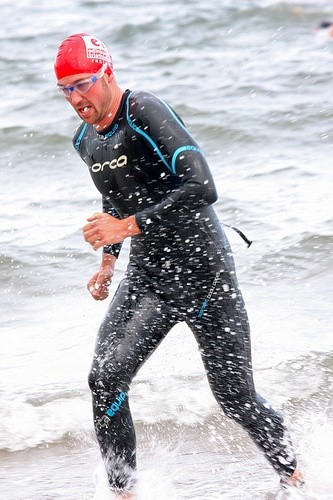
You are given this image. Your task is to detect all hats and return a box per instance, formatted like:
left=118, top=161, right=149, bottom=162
left=54, top=34, right=113, bottom=81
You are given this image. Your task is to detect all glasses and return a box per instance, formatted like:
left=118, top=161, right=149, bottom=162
left=59, top=63, right=104, bottom=99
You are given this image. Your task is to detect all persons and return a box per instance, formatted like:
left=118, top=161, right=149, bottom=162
left=52, top=33, right=310, bottom=498
